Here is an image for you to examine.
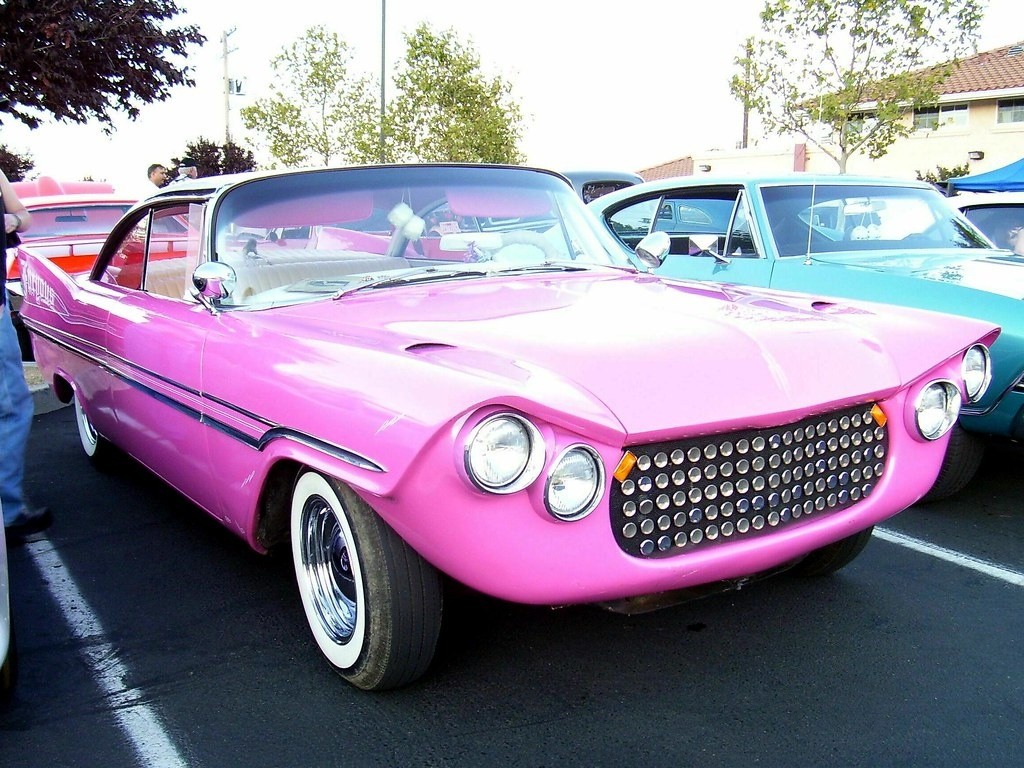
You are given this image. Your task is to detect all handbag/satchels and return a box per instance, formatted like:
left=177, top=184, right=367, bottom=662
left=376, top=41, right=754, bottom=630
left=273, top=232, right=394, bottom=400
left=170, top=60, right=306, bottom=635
left=11, top=311, right=36, bottom=362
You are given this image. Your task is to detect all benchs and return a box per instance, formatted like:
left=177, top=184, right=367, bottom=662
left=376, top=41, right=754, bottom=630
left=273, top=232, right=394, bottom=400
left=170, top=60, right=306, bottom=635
left=146, top=248, right=411, bottom=300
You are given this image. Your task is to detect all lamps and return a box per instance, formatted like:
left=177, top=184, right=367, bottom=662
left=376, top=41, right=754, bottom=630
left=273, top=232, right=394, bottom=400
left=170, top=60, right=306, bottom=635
left=699, top=164, right=712, bottom=172
left=969, top=151, right=985, bottom=160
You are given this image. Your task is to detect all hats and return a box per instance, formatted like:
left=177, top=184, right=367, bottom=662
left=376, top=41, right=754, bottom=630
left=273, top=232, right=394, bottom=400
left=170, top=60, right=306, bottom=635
left=179, top=158, right=202, bottom=167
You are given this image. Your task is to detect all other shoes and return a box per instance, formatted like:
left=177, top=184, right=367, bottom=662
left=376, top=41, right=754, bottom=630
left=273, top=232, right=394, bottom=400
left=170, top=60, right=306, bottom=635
left=5, top=507, right=53, bottom=535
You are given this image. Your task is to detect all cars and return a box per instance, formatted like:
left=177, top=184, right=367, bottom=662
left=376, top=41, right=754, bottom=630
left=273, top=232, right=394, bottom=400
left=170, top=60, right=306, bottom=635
left=225, top=165, right=846, bottom=267
left=6, top=177, right=199, bottom=350
left=20, top=162, right=999, bottom=683
left=538, top=171, right=1024, bottom=475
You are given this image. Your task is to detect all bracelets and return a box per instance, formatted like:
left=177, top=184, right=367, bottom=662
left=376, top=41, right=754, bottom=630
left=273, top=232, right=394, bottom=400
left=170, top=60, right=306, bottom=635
left=13, top=213, right=22, bottom=230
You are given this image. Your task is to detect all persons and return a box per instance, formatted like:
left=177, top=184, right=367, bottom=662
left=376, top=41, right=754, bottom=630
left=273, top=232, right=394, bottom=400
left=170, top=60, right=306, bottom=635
left=123, top=158, right=198, bottom=243
left=0, top=172, right=56, bottom=546
